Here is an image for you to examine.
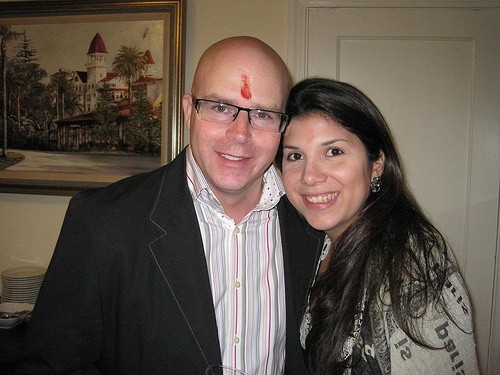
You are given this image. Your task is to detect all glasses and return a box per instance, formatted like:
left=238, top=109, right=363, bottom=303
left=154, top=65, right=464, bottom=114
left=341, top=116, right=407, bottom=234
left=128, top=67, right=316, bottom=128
left=190, top=93, right=290, bottom=133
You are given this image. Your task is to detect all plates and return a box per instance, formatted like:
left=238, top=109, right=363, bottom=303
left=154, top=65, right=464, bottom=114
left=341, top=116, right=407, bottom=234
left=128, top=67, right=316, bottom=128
left=1, top=266, right=46, bottom=303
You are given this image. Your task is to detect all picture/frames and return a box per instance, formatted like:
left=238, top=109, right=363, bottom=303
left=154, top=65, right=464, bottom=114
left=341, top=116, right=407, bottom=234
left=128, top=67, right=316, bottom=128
left=0, top=0, right=187, bottom=196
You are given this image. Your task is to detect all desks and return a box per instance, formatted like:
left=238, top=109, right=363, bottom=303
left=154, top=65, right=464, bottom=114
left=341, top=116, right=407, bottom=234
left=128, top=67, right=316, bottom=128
left=1, top=319, right=29, bottom=375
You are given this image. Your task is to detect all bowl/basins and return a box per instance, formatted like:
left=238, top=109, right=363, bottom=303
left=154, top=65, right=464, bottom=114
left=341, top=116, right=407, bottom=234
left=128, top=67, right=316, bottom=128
left=0, top=302, right=35, bottom=330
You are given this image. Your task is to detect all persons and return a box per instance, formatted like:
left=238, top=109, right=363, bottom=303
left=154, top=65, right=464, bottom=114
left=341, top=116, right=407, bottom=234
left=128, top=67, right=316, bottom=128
left=277, top=77, right=479, bottom=375
left=14, top=35, right=324, bottom=375
